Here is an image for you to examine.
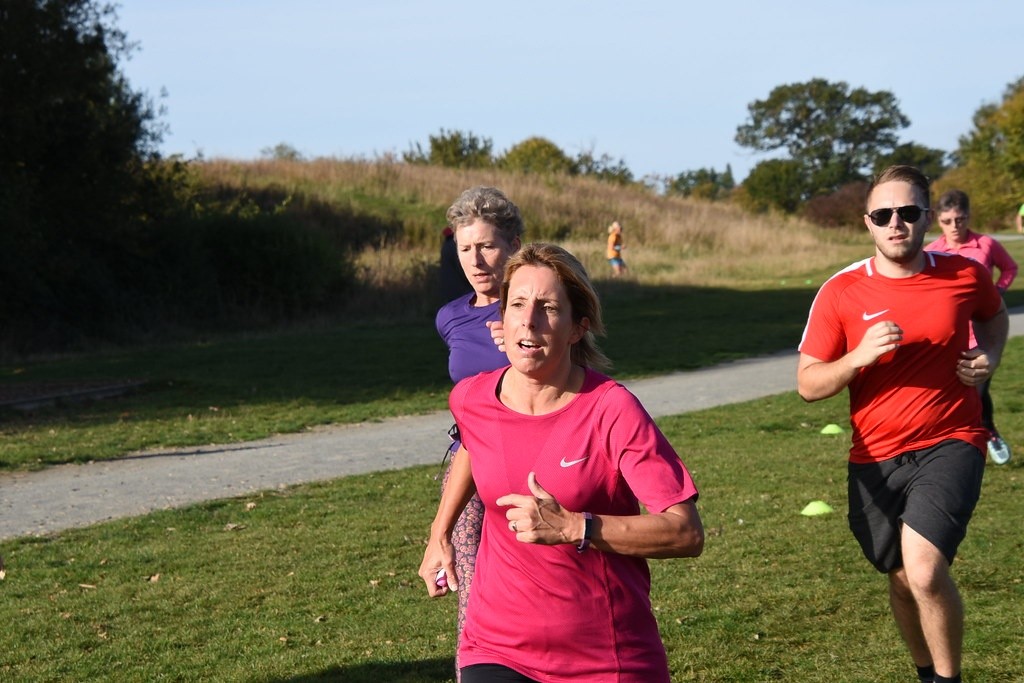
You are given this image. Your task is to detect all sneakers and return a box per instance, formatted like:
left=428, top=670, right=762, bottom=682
left=988, top=436, right=1010, bottom=464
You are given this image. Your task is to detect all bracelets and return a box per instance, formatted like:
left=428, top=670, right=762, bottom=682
left=576, top=511, right=592, bottom=553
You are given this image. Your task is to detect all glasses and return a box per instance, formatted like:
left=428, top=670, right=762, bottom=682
left=868, top=205, right=929, bottom=226
left=941, top=216, right=968, bottom=225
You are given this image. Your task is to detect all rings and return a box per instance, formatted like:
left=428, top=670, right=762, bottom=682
left=512, top=522, right=518, bottom=531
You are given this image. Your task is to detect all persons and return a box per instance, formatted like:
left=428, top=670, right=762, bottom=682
left=606, top=222, right=628, bottom=279
left=436, top=186, right=525, bottom=683
left=417, top=241, right=704, bottom=682
left=794, top=165, right=1010, bottom=683
left=1016, top=204, right=1024, bottom=235
left=919, top=189, right=1010, bottom=465
